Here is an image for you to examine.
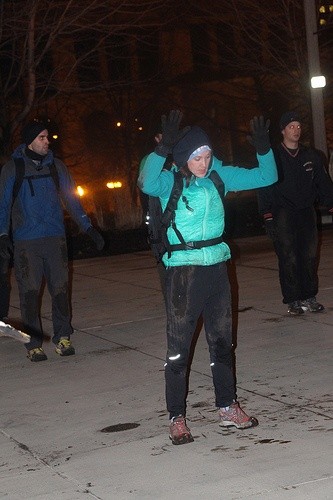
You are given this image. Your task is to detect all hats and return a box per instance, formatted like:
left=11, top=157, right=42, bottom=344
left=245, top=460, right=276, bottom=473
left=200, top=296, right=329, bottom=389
left=173, top=126, right=212, bottom=169
left=24, top=123, right=46, bottom=144
left=279, top=112, right=302, bottom=130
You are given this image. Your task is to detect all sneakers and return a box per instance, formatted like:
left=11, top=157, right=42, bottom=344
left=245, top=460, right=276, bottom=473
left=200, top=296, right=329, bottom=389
left=168, top=414, right=194, bottom=445
left=55, top=336, right=75, bottom=355
left=218, top=404, right=258, bottom=429
left=287, top=301, right=304, bottom=314
left=27, top=348, right=48, bottom=362
left=299, top=297, right=324, bottom=312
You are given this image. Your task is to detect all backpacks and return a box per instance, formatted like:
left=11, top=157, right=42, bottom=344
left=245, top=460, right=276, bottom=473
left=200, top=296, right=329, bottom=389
left=146, top=170, right=226, bottom=264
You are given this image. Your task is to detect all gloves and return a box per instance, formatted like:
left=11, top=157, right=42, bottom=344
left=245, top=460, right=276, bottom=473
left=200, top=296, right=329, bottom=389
left=247, top=115, right=271, bottom=155
left=265, top=220, right=275, bottom=239
left=155, top=109, right=191, bottom=157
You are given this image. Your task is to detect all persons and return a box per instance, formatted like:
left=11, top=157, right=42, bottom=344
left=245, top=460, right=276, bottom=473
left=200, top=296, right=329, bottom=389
left=0, top=123, right=104, bottom=363
left=260, top=110, right=333, bottom=316
left=138, top=109, right=279, bottom=445
